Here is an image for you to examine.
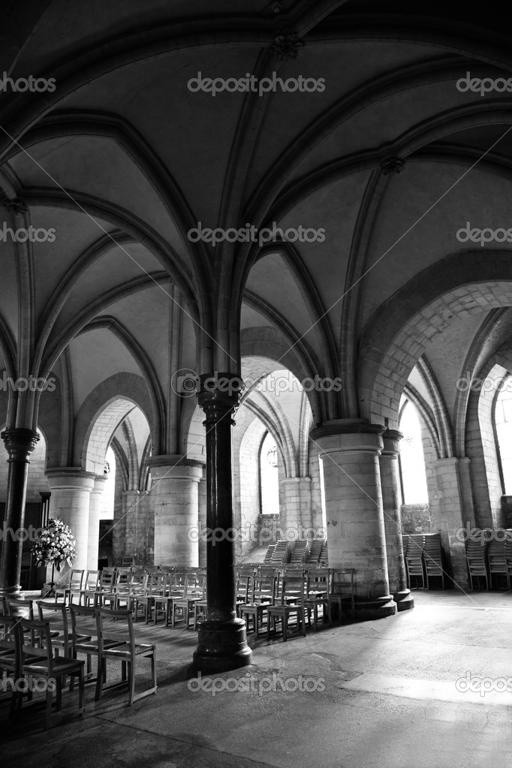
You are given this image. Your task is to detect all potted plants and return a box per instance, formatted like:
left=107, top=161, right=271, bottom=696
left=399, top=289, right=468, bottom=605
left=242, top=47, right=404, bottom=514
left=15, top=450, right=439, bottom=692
left=32, top=518, right=77, bottom=597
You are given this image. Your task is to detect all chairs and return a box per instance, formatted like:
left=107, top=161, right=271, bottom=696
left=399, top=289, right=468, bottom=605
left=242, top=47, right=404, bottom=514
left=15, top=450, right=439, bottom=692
left=0, top=598, right=151, bottom=724
left=465, top=528, right=511, bottom=590
left=264, top=534, right=445, bottom=588
left=55, top=568, right=357, bottom=641
